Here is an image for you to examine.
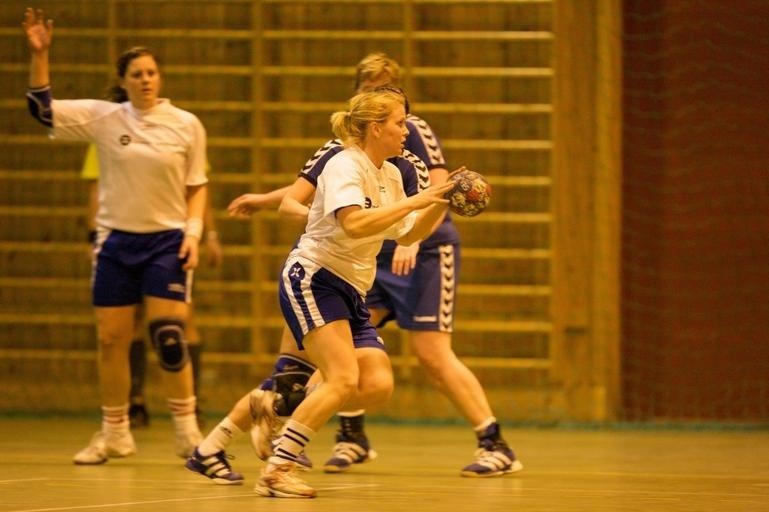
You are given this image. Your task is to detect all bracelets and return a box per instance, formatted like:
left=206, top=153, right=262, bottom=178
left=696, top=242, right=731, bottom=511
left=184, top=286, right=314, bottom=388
left=206, top=231, right=221, bottom=240
left=185, top=217, right=205, bottom=241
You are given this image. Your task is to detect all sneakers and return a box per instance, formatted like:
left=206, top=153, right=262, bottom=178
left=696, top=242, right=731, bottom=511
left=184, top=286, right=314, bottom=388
left=178, top=409, right=314, bottom=471
left=458, top=445, right=524, bottom=479
left=182, top=446, right=246, bottom=486
left=71, top=426, right=137, bottom=466
left=253, top=465, right=318, bottom=500
left=320, top=430, right=380, bottom=474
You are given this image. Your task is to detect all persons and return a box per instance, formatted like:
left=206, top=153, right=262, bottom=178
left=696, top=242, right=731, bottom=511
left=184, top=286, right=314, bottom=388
left=246, top=90, right=470, bottom=499
left=227, top=48, right=526, bottom=479
left=18, top=3, right=212, bottom=465
left=184, top=81, right=431, bottom=487
left=77, top=84, right=224, bottom=430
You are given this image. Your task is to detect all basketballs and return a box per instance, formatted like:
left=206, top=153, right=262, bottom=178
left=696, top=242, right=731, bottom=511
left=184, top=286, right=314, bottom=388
left=443, top=171, right=492, bottom=218
